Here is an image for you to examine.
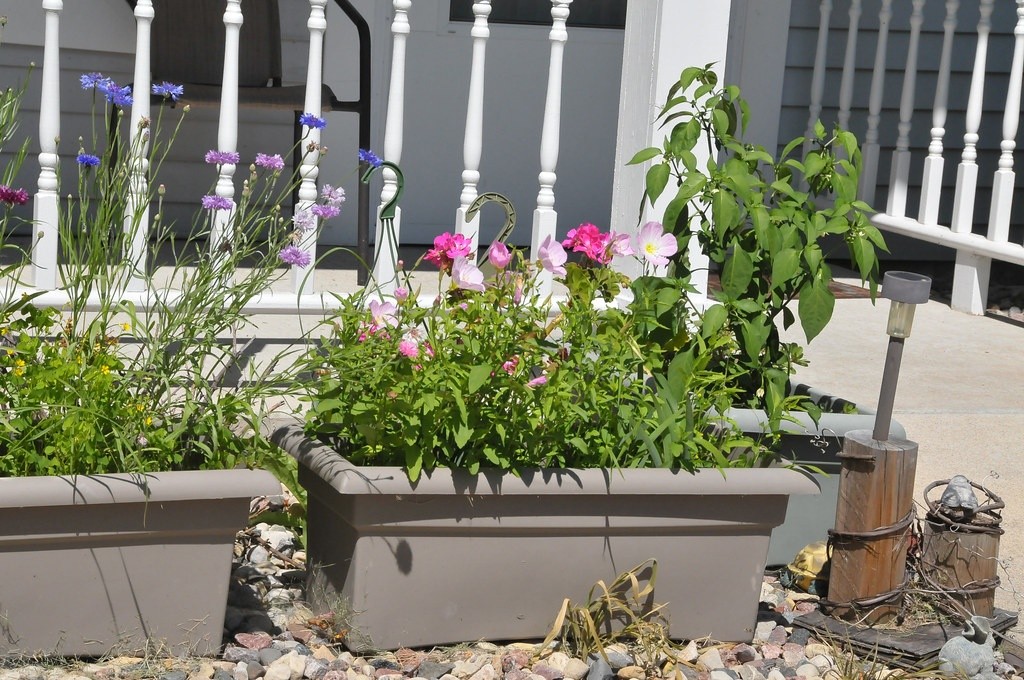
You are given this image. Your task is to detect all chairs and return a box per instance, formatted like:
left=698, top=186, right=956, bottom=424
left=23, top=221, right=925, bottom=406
left=107, top=0, right=375, bottom=287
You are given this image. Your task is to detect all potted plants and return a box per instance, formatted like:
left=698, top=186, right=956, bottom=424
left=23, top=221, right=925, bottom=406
left=627, top=61, right=911, bottom=577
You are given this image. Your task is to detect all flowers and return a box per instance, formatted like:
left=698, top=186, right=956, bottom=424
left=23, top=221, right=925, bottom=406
left=0, top=66, right=391, bottom=514
left=269, top=217, right=838, bottom=486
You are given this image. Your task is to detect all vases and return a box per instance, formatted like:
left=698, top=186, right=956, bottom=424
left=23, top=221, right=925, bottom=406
left=267, top=417, right=826, bottom=658
left=0, top=470, right=288, bottom=665
